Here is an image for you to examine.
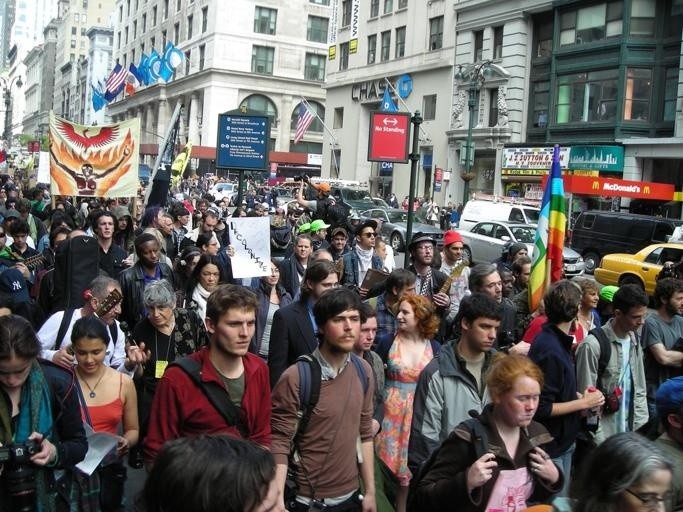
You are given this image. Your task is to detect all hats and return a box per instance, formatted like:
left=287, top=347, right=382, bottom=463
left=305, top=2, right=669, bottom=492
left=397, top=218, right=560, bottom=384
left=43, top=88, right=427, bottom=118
left=354, top=218, right=378, bottom=235
left=298, top=220, right=349, bottom=240
left=509, top=243, right=528, bottom=258
left=4, top=210, right=20, bottom=219
left=442, top=230, right=464, bottom=247
left=407, top=231, right=436, bottom=252
left=314, top=182, right=331, bottom=193
left=656, top=375, right=683, bottom=409
left=0, top=269, right=31, bottom=305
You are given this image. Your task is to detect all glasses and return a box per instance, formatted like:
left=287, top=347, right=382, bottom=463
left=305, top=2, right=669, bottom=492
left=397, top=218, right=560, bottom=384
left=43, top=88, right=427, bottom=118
left=625, top=488, right=674, bottom=506
left=362, top=233, right=377, bottom=237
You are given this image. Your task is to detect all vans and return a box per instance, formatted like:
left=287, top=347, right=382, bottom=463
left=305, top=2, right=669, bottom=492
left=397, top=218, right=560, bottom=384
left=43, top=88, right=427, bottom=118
left=569, top=208, right=683, bottom=275
left=459, top=198, right=542, bottom=237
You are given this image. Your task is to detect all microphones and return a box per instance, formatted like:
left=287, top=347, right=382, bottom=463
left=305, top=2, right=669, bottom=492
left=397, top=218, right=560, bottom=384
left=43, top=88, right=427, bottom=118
left=120, top=321, right=140, bottom=349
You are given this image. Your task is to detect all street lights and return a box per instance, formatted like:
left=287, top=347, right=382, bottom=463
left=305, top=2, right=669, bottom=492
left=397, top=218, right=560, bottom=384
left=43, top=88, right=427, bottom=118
left=0, top=74, right=24, bottom=142
left=453, top=59, right=500, bottom=209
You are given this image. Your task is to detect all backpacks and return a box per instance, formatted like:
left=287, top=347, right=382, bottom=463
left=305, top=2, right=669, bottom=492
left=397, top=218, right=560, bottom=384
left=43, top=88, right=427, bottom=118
left=406, top=418, right=545, bottom=512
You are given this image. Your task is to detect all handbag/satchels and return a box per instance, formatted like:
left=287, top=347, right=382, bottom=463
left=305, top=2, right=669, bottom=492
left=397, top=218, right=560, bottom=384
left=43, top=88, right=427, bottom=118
left=99, top=463, right=127, bottom=510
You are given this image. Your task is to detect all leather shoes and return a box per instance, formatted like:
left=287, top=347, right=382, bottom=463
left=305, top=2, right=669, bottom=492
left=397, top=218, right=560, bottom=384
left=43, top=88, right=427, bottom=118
left=130, top=448, right=144, bottom=469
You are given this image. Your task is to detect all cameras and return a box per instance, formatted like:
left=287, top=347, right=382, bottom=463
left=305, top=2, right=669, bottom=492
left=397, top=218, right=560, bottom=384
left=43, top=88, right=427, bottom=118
left=294, top=172, right=308, bottom=182
left=0, top=440, right=37, bottom=496
left=308, top=499, right=327, bottom=512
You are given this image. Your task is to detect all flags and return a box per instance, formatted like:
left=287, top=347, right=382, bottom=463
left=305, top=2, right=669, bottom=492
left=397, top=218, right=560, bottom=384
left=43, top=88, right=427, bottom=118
left=293, top=100, right=316, bottom=146
left=91, top=42, right=185, bottom=112
left=381, top=85, right=398, bottom=114
left=144, top=102, right=180, bottom=214
left=527, top=142, right=565, bottom=320
left=168, top=142, right=191, bottom=187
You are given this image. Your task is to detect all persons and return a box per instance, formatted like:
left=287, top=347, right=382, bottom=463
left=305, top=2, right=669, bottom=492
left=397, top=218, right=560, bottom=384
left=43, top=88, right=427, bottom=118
left=0, top=159, right=682, bottom=512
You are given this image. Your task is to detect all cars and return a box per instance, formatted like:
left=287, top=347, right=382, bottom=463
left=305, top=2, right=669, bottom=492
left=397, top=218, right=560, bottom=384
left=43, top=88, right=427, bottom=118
left=452, top=219, right=584, bottom=283
left=357, top=203, right=447, bottom=252
left=136, top=163, right=155, bottom=185
left=372, top=196, right=394, bottom=210
left=591, top=242, right=683, bottom=293
left=274, top=187, right=293, bottom=206
left=208, top=179, right=239, bottom=207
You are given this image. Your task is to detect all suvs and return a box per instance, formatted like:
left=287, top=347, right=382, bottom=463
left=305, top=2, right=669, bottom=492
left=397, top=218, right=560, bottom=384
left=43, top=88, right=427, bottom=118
left=331, top=183, right=378, bottom=221
